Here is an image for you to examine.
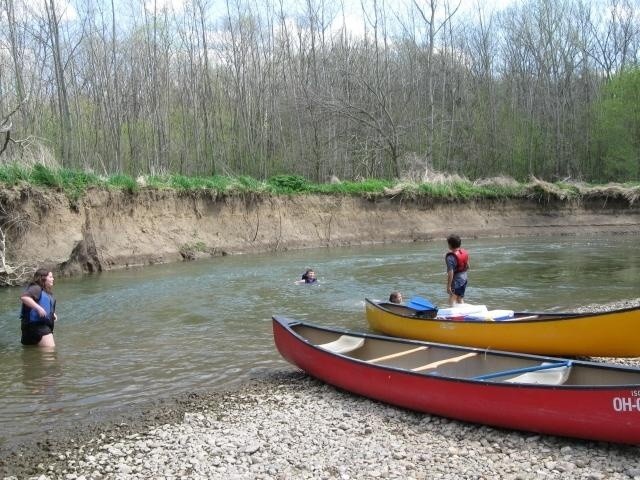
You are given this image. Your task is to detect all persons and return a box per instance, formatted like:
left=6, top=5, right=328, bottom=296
left=20, top=268, right=57, bottom=348
left=444, top=233, right=471, bottom=309
left=291, top=269, right=320, bottom=285
left=387, top=292, right=405, bottom=305
left=22, top=348, right=64, bottom=424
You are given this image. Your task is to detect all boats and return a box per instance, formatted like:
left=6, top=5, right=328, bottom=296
left=365, top=298, right=640, bottom=357
left=272, top=315, right=640, bottom=446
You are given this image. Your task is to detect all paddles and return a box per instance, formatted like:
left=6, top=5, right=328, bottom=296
left=407, top=296, right=439, bottom=312
